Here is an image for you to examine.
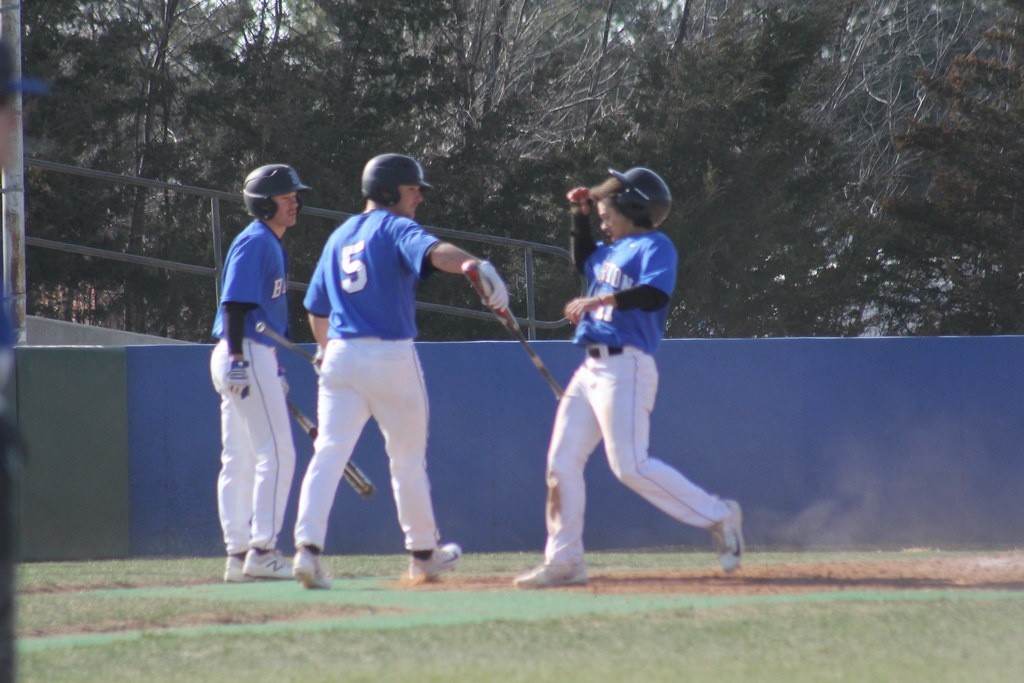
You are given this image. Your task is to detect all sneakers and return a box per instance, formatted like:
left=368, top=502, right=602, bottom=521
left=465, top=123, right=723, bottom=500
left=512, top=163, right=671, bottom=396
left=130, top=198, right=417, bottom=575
left=409, top=542, right=462, bottom=579
left=293, top=547, right=331, bottom=589
left=224, top=556, right=254, bottom=581
left=710, top=500, right=745, bottom=573
left=242, top=548, right=294, bottom=579
left=514, top=559, right=591, bottom=589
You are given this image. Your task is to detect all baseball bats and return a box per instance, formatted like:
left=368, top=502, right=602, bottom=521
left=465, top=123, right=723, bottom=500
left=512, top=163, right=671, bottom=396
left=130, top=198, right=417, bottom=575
left=256, top=322, right=322, bottom=366
left=460, top=258, right=562, bottom=399
left=284, top=399, right=376, bottom=500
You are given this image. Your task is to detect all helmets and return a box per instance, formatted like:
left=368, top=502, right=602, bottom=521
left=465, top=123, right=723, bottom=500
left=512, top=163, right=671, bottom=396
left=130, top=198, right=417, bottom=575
left=608, top=166, right=672, bottom=228
left=243, top=163, right=312, bottom=221
left=362, top=153, right=433, bottom=207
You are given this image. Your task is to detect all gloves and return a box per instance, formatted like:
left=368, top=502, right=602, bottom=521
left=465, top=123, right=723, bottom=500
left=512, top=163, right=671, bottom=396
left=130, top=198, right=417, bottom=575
left=479, top=261, right=509, bottom=313
left=278, top=367, right=289, bottom=395
left=313, top=345, right=325, bottom=375
left=223, top=360, right=253, bottom=400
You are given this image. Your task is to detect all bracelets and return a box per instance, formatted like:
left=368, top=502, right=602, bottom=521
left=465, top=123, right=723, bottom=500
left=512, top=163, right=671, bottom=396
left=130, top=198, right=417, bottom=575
left=598, top=297, right=603, bottom=304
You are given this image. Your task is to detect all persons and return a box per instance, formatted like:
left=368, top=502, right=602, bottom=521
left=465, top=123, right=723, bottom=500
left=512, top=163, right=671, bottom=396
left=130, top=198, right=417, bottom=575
left=0, top=44, right=53, bottom=683
left=291, top=152, right=510, bottom=590
left=210, top=163, right=312, bottom=583
left=511, top=168, right=742, bottom=589
